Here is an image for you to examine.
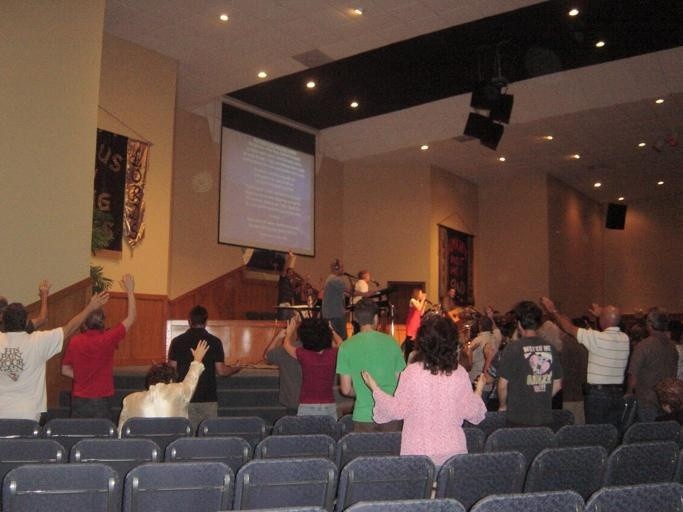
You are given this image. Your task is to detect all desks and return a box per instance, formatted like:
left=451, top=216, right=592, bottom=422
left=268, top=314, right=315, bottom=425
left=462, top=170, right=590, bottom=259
left=264, top=304, right=353, bottom=361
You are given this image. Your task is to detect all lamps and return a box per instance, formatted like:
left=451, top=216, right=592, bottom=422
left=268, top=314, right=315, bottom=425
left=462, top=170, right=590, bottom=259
left=463, top=77, right=514, bottom=150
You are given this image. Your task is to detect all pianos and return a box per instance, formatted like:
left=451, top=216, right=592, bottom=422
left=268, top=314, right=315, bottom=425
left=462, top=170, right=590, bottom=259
left=368, top=285, right=398, bottom=298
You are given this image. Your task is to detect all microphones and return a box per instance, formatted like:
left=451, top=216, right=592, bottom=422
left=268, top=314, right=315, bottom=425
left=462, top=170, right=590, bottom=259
left=425, top=298, right=433, bottom=304
left=370, top=279, right=381, bottom=285
left=344, top=272, right=357, bottom=279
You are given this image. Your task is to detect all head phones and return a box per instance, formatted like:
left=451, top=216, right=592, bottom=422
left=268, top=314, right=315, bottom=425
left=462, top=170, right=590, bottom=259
left=335, top=259, right=340, bottom=270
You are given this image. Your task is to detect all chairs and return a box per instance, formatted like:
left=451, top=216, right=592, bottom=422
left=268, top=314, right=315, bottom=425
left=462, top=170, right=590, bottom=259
left=0, top=409, right=683, bottom=512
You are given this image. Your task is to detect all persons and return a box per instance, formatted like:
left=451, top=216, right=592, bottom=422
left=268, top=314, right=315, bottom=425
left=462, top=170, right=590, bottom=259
left=361, top=315, right=486, bottom=466
left=61, top=274, right=137, bottom=419
left=0, top=279, right=51, bottom=334
left=265, top=249, right=427, bottom=421
left=0, top=291, right=110, bottom=439
left=443, top=288, right=681, bottom=424
left=152, top=305, right=247, bottom=437
left=117, top=340, right=210, bottom=439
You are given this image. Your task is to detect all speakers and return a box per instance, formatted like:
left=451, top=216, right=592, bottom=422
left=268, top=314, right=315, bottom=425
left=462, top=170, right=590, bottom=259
left=387, top=281, right=425, bottom=324
left=463, top=41, right=514, bottom=150
